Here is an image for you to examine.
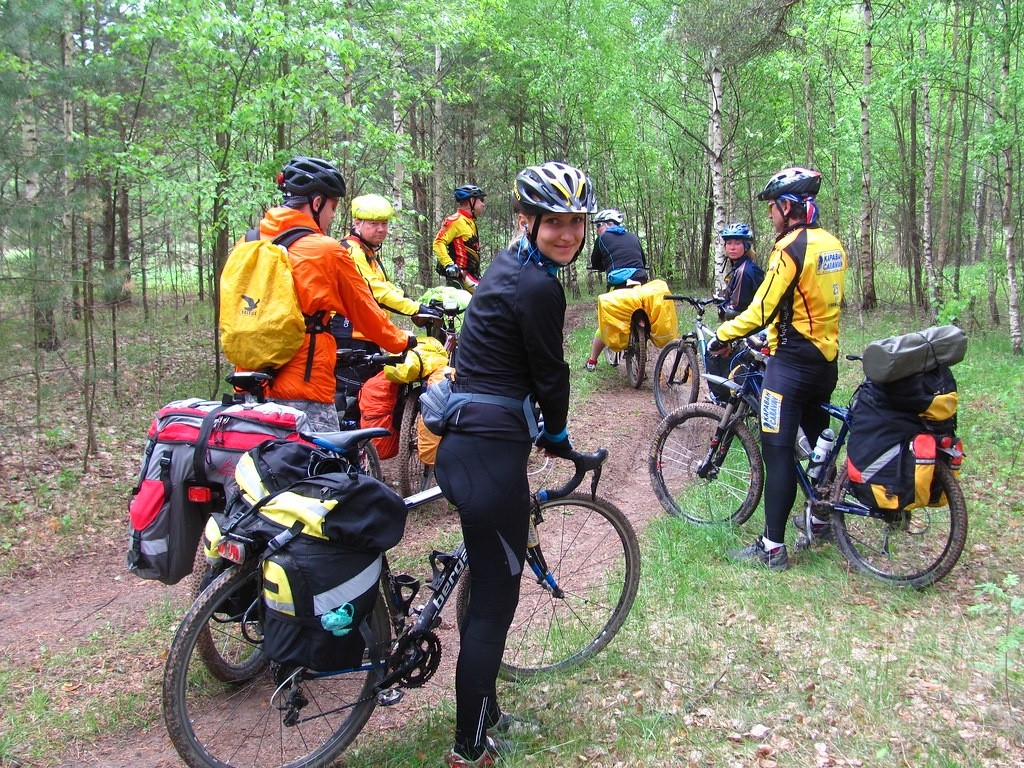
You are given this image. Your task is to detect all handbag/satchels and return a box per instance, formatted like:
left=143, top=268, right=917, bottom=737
left=126, top=336, right=538, bottom=679
left=703, top=332, right=769, bottom=415
left=417, top=286, right=472, bottom=346
left=597, top=268, right=679, bottom=353
left=847, top=324, right=969, bottom=513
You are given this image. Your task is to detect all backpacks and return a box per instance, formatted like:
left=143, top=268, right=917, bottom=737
left=218, top=227, right=317, bottom=372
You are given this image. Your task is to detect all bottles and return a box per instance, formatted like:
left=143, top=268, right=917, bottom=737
left=806, top=429, right=834, bottom=479
left=794, top=425, right=812, bottom=459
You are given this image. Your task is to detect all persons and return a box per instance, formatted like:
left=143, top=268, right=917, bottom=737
left=584, top=208, right=648, bottom=372
left=234, top=157, right=417, bottom=434
left=432, top=184, right=488, bottom=296
left=433, top=160, right=599, bottom=768
left=707, top=166, right=848, bottom=570
left=703, top=222, right=770, bottom=408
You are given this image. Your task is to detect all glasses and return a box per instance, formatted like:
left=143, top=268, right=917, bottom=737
left=597, top=222, right=610, bottom=229
left=476, top=196, right=484, bottom=202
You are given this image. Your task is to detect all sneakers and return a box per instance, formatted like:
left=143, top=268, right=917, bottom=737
left=793, top=512, right=836, bottom=544
left=586, top=358, right=597, bottom=372
left=485, top=714, right=543, bottom=740
left=447, top=735, right=523, bottom=768
left=729, top=535, right=789, bottom=573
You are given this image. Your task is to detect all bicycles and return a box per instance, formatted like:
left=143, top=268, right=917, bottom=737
left=646, top=329, right=969, bottom=591
left=585, top=264, right=652, bottom=389
left=652, top=292, right=769, bottom=455
left=189, top=332, right=417, bottom=687
left=393, top=263, right=473, bottom=509
left=161, top=411, right=643, bottom=768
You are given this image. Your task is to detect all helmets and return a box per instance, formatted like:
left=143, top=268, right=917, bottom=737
left=591, top=209, right=622, bottom=224
left=721, top=223, right=753, bottom=239
left=757, top=167, right=822, bottom=202
left=277, top=157, right=346, bottom=197
left=455, top=185, right=487, bottom=203
left=510, top=162, right=597, bottom=214
left=352, top=194, right=394, bottom=220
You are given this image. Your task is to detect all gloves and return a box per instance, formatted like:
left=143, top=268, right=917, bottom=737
left=418, top=304, right=443, bottom=317
left=446, top=264, right=460, bottom=277
left=406, top=335, right=417, bottom=350
